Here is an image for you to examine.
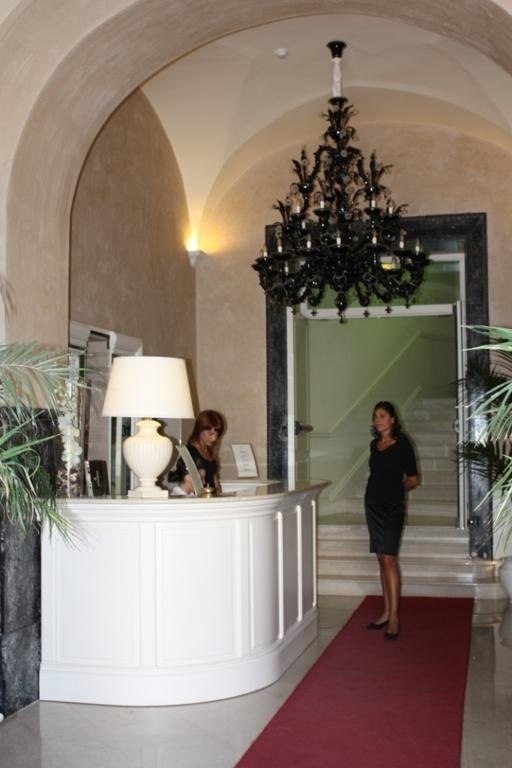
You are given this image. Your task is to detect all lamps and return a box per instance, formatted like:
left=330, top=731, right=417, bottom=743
left=99, top=355, right=198, bottom=499
left=252, top=40, right=431, bottom=321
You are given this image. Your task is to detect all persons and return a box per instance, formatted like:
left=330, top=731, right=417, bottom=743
left=167, top=408, right=225, bottom=492
left=363, top=399, right=421, bottom=641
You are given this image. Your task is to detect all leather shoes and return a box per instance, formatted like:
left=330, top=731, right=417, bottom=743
left=384, top=622, right=399, bottom=639
left=366, top=617, right=388, bottom=632
